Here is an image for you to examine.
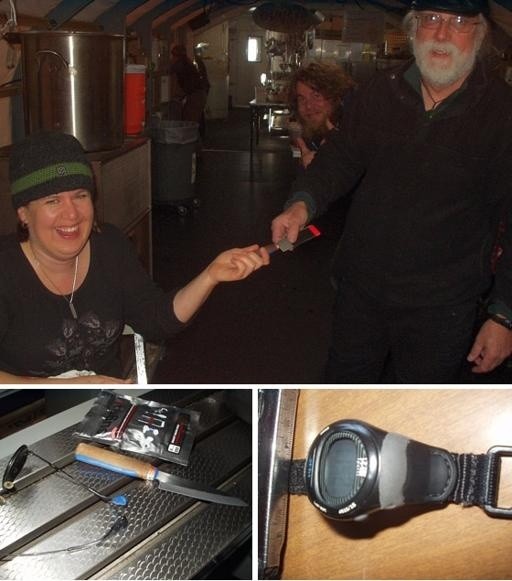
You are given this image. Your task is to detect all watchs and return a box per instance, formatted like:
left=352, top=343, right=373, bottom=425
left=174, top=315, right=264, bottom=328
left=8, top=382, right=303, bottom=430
left=286, top=414, right=512, bottom=536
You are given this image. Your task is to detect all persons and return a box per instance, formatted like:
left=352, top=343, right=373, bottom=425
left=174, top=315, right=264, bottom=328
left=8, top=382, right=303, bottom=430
left=2, top=130, right=272, bottom=385
left=264, top=0, right=510, bottom=383
left=164, top=42, right=207, bottom=125
left=286, top=62, right=344, bottom=168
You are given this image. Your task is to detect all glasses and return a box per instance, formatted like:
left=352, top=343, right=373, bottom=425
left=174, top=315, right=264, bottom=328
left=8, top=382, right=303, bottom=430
left=414, top=14, right=484, bottom=35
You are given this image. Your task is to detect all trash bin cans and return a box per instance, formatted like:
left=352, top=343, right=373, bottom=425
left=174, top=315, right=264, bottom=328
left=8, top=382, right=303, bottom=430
left=147, top=121, right=202, bottom=216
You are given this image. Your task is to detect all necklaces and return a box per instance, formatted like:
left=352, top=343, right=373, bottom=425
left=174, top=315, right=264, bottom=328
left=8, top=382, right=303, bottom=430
left=27, top=242, right=81, bottom=320
left=420, top=81, right=446, bottom=125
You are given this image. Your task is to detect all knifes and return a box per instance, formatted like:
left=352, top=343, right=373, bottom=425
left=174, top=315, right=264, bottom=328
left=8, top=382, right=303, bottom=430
left=74, top=442, right=251, bottom=507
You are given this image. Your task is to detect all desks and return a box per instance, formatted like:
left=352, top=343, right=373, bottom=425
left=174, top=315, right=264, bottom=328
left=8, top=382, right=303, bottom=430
left=248, top=96, right=295, bottom=168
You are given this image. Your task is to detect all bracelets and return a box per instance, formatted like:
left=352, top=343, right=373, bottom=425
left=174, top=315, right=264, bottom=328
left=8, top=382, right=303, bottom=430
left=488, top=312, right=510, bottom=331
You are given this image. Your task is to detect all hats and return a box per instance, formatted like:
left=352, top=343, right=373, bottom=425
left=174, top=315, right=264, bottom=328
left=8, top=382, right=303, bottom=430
left=9, top=130, right=98, bottom=211
left=409, top=1, right=491, bottom=17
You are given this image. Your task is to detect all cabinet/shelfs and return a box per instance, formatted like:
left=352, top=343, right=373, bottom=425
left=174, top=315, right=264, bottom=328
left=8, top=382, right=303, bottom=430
left=82, top=135, right=154, bottom=283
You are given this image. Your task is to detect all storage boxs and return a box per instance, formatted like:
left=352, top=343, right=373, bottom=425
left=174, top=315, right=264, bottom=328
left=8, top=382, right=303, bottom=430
left=124, top=56, right=147, bottom=133
